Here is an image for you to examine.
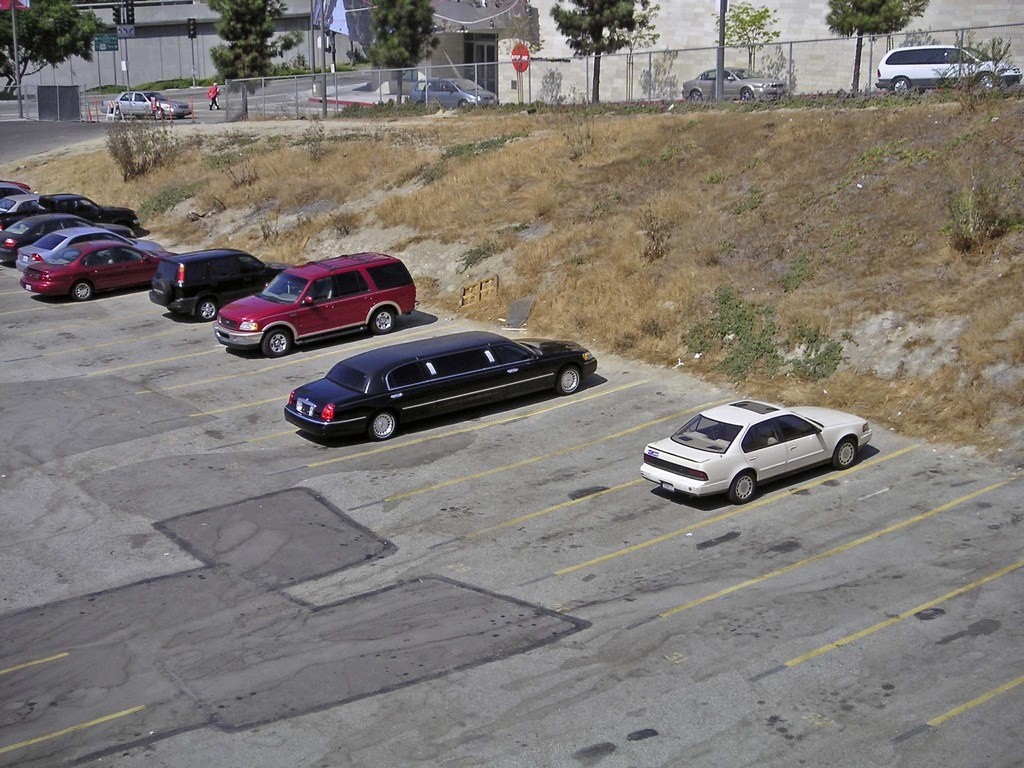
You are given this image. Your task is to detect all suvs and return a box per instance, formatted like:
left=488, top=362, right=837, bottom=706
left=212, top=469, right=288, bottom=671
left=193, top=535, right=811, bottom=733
left=149, top=248, right=295, bottom=322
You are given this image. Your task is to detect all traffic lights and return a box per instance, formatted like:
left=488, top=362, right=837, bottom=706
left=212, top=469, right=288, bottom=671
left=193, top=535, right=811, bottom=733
left=112, top=5, right=121, bottom=24
left=126, top=0, right=135, bottom=24
left=187, top=18, right=196, bottom=38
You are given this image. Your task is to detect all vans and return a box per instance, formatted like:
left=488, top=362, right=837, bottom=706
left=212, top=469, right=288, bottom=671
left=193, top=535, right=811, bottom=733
left=875, top=45, right=1024, bottom=96
left=410, top=78, right=498, bottom=116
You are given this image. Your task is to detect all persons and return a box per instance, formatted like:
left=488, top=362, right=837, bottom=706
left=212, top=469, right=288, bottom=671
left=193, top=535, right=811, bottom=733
left=208, top=83, right=220, bottom=110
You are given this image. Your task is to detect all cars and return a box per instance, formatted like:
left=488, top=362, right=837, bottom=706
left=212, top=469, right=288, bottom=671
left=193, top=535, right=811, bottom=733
left=640, top=400, right=872, bottom=505
left=0, top=213, right=137, bottom=265
left=284, top=331, right=598, bottom=442
left=0, top=180, right=42, bottom=217
left=16, top=226, right=164, bottom=272
left=681, top=67, right=785, bottom=104
left=20, top=241, right=181, bottom=302
left=101, top=91, right=191, bottom=119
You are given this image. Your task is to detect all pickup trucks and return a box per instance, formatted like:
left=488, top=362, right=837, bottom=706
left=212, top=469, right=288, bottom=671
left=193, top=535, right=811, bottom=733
left=212, top=252, right=420, bottom=359
left=0, top=194, right=140, bottom=236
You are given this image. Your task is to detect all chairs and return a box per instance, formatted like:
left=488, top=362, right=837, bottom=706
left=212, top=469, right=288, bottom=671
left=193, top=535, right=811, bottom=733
left=753, top=426, right=772, bottom=447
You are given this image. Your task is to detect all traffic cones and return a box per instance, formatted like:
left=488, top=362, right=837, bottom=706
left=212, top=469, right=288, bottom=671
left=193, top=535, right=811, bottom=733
left=87, top=105, right=92, bottom=123
left=94, top=97, right=99, bottom=122
left=167, top=96, right=174, bottom=122
left=190, top=94, right=194, bottom=122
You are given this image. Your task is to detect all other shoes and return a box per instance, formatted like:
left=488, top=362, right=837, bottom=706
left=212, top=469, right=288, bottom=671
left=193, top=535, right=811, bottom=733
left=217, top=108, right=220, bottom=110
left=210, top=108, right=212, bottom=110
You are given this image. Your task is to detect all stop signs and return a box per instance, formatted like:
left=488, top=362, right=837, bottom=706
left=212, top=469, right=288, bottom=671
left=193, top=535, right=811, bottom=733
left=511, top=44, right=530, bottom=72
